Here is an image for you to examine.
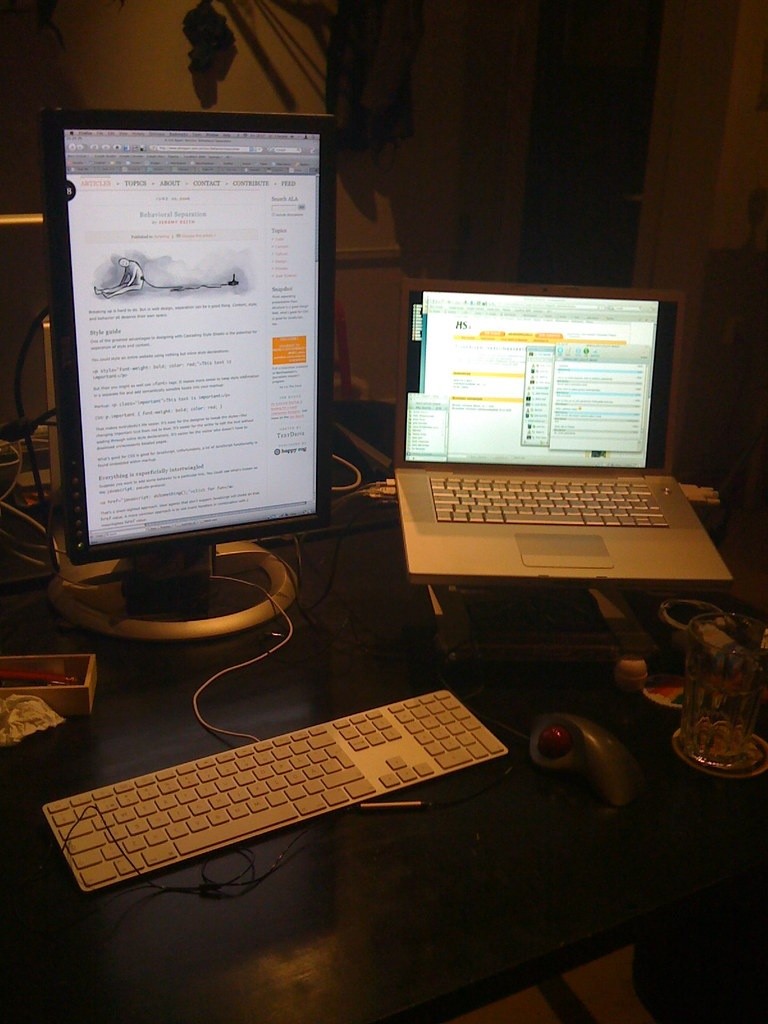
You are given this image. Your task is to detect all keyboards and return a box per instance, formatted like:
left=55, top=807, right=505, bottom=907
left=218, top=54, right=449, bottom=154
left=41, top=690, right=509, bottom=892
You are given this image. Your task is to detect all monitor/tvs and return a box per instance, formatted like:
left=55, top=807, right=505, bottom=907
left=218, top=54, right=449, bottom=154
left=37, top=106, right=339, bottom=640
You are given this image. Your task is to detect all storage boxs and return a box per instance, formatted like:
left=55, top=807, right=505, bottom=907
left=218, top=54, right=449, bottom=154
left=0, top=654, right=98, bottom=716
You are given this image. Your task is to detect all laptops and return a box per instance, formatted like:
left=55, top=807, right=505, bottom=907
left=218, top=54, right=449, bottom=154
left=393, top=281, right=734, bottom=594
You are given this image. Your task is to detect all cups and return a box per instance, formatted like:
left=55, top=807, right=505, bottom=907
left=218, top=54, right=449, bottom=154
left=683, top=612, right=768, bottom=765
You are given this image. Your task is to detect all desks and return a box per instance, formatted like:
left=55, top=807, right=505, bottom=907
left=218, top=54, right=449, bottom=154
left=0, top=398, right=768, bottom=1024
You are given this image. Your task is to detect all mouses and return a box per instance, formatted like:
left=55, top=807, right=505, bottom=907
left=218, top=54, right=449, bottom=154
left=528, top=712, right=648, bottom=808
